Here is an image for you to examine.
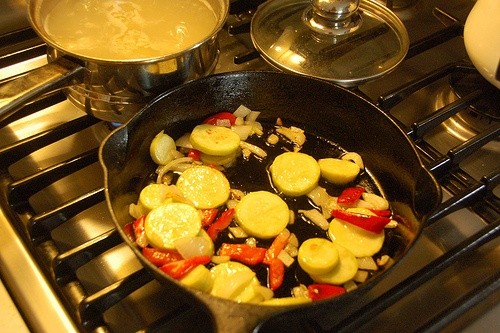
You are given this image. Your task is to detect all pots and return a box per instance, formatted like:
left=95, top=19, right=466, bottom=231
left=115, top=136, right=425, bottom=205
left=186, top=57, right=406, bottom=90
left=0, top=0, right=230, bottom=122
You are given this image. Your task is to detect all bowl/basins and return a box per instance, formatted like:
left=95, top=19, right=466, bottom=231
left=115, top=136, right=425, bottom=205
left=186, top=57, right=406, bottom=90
left=99, top=68, right=443, bottom=320
